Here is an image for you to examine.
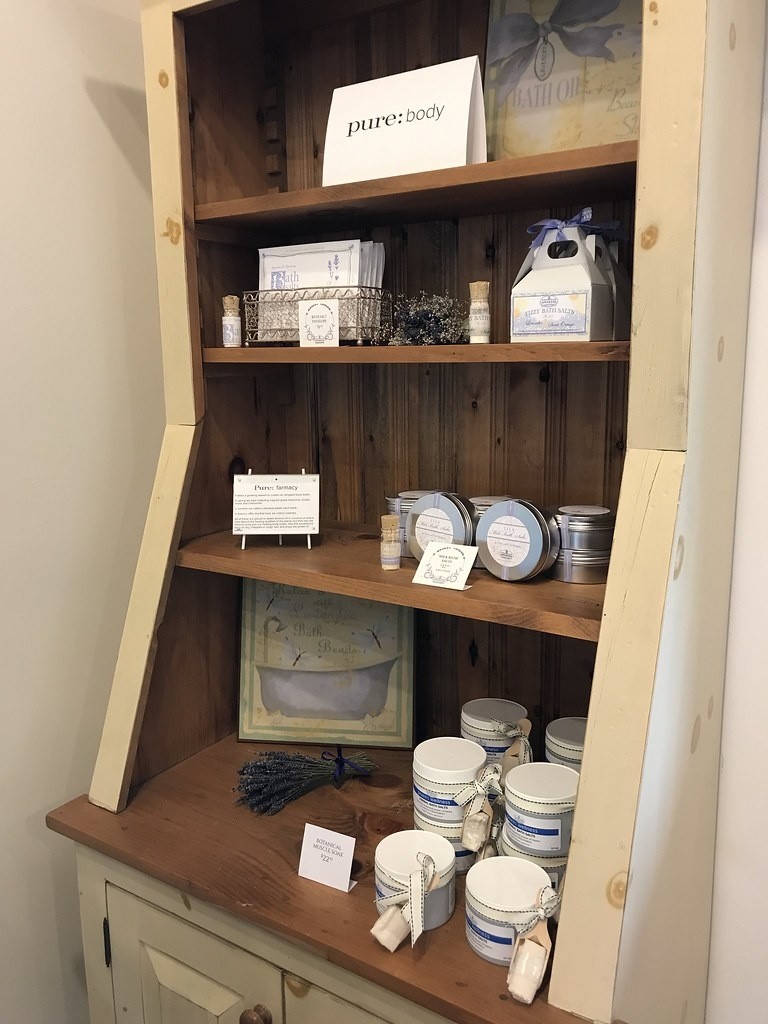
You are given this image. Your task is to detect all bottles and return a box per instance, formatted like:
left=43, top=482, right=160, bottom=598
left=222, top=294, right=242, bottom=348
left=469, top=281, right=491, bottom=343
left=379, top=514, right=402, bottom=571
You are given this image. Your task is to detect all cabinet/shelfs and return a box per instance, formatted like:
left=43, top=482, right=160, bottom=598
left=41, top=0, right=768, bottom=1024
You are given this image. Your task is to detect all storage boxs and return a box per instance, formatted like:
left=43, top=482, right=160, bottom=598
left=242, top=283, right=394, bottom=344
